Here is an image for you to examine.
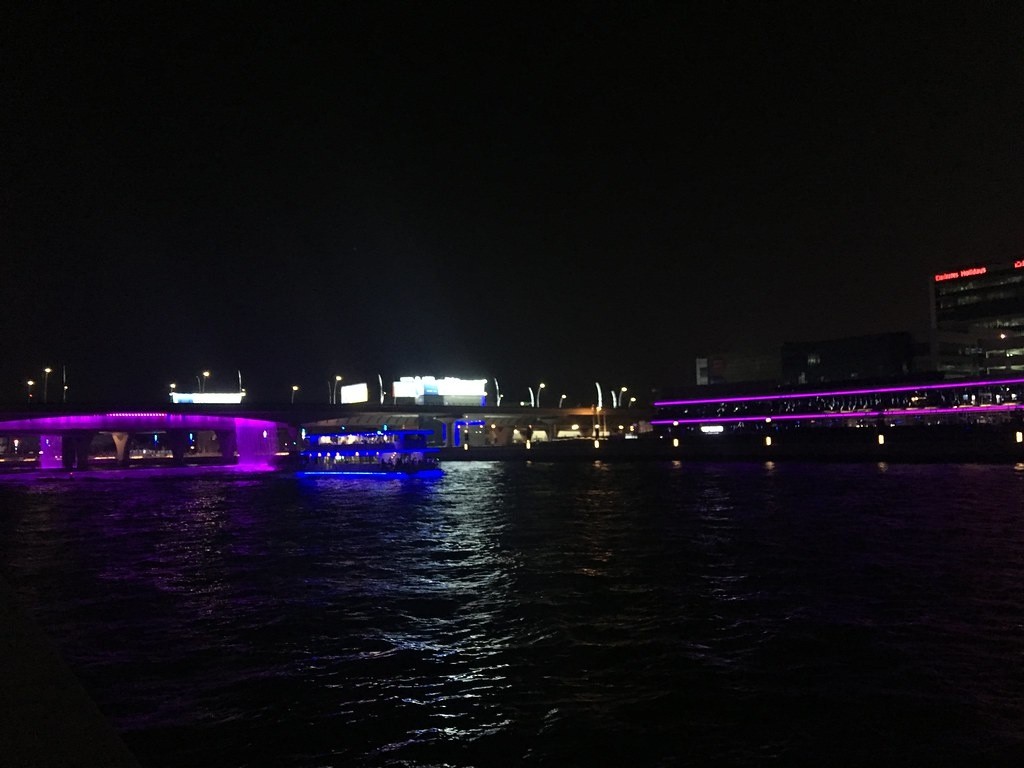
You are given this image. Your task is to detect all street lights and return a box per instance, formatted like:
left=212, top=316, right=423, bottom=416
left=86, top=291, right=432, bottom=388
left=490, top=374, right=637, bottom=409
left=167, top=369, right=248, bottom=402
left=289, top=371, right=389, bottom=407
left=24, top=363, right=67, bottom=402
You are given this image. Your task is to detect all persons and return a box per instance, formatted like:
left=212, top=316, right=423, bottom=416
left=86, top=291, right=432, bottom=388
left=381, top=458, right=435, bottom=470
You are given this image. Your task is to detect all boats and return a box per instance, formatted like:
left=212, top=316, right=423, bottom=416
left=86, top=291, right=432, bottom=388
left=298, top=424, right=440, bottom=476
